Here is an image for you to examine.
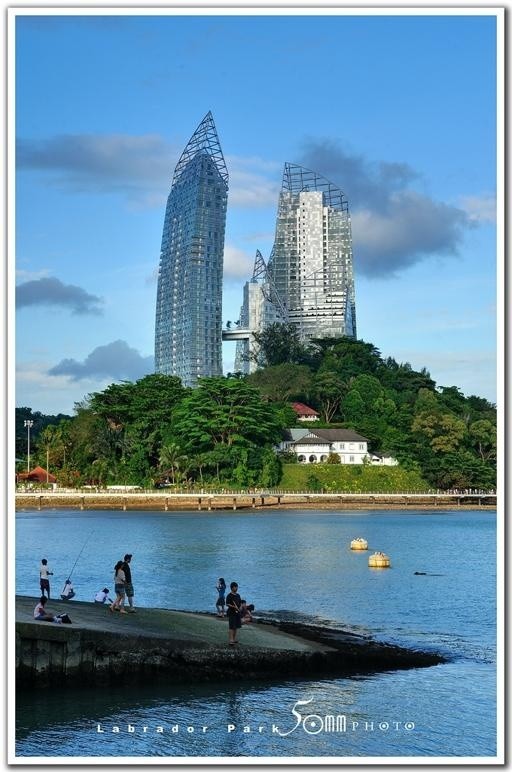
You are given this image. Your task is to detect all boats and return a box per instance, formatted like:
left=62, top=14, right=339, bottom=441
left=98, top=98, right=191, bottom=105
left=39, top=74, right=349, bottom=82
left=368, top=551, right=391, bottom=567
left=351, top=538, right=368, bottom=550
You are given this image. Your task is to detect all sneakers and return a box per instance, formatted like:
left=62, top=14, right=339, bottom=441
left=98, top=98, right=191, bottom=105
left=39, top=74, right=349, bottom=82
left=110, top=606, right=135, bottom=614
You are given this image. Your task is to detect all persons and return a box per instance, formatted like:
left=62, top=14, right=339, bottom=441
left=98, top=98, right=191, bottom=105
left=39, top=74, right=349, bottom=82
left=226, top=582, right=242, bottom=646
left=60, top=580, right=75, bottom=600
left=114, top=554, right=137, bottom=612
left=39, top=559, right=54, bottom=599
left=95, top=588, right=114, bottom=605
left=215, top=578, right=225, bottom=618
left=109, top=561, right=128, bottom=614
left=239, top=600, right=252, bottom=624
left=245, top=605, right=254, bottom=617
left=34, top=596, right=53, bottom=621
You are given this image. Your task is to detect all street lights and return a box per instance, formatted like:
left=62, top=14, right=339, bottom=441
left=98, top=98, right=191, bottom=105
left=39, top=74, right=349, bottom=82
left=24, top=419, right=34, bottom=473
left=62, top=443, right=68, bottom=468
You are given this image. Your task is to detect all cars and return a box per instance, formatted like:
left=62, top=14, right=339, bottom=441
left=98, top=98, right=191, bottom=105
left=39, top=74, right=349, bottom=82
left=155, top=480, right=173, bottom=489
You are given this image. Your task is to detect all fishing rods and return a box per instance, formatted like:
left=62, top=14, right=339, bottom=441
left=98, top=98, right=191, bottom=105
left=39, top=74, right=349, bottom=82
left=232, top=600, right=263, bottom=652
left=68, top=529, right=95, bottom=579
